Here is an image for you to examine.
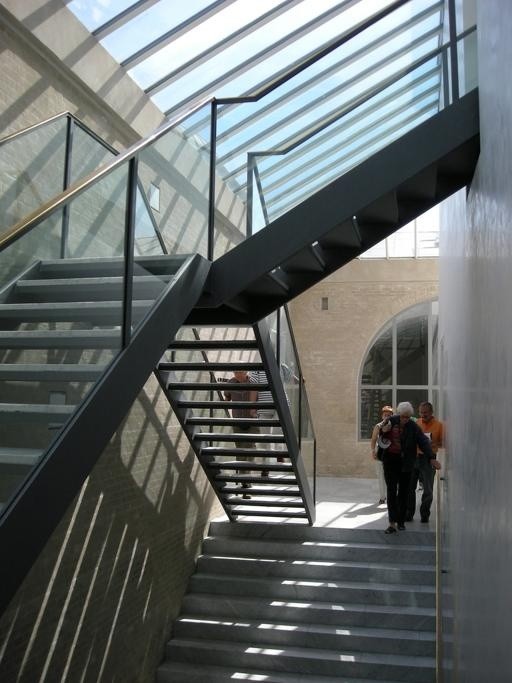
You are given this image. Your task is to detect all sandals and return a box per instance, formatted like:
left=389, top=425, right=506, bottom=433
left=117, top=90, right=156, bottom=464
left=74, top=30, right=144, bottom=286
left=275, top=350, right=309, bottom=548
left=384, top=525, right=405, bottom=534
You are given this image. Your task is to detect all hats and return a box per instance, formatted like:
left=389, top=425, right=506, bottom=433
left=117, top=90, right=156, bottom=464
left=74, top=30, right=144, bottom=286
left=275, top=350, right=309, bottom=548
left=382, top=406, right=393, bottom=412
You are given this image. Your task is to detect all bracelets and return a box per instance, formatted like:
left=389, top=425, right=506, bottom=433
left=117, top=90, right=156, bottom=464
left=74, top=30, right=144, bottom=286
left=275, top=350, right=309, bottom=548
left=371, top=449, right=375, bottom=453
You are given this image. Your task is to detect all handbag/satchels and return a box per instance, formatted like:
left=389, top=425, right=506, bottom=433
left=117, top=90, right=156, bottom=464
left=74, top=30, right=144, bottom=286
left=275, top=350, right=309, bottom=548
left=375, top=435, right=392, bottom=462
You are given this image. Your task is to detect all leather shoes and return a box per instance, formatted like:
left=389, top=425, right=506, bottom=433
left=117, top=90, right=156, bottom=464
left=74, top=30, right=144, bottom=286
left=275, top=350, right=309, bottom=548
left=240, top=483, right=252, bottom=499
left=379, top=497, right=386, bottom=504
left=407, top=515, right=429, bottom=523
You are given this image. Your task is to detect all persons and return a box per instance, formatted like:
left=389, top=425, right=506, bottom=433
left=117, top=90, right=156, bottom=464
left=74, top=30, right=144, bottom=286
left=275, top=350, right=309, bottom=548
left=223, top=360, right=259, bottom=499
left=249, top=342, right=306, bottom=477
left=375, top=402, right=442, bottom=536
left=405, top=401, right=443, bottom=522
left=370, top=405, right=396, bottom=504
